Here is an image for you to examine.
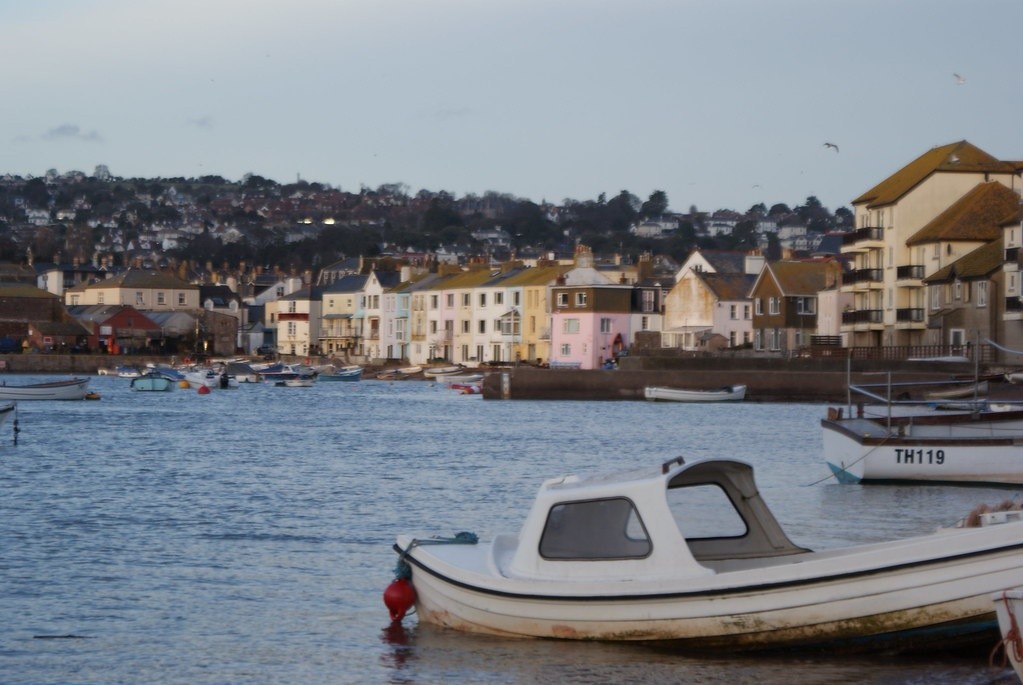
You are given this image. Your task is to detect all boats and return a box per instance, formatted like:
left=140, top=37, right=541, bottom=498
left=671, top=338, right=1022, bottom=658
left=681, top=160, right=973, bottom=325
left=384, top=453, right=1023, bottom=646
left=644, top=383, right=747, bottom=403
left=819, top=329, right=1023, bottom=486
left=423, top=363, right=467, bottom=381
left=0, top=401, right=18, bottom=414
left=435, top=373, right=484, bottom=387
left=374, top=365, right=423, bottom=382
left=97, top=356, right=365, bottom=394
left=0, top=377, right=102, bottom=402
